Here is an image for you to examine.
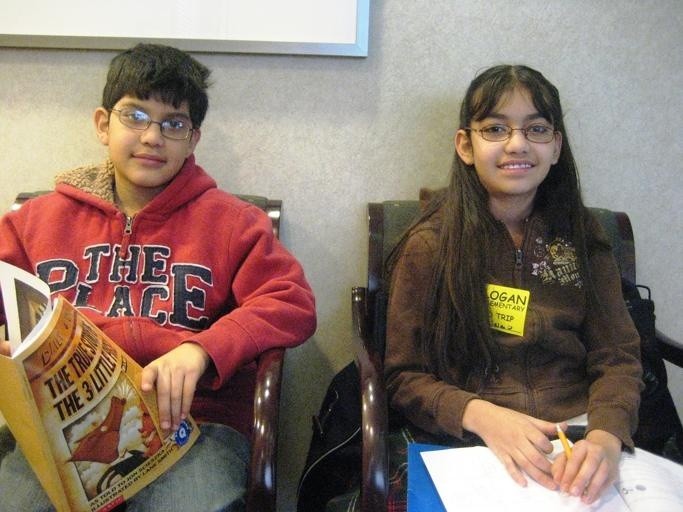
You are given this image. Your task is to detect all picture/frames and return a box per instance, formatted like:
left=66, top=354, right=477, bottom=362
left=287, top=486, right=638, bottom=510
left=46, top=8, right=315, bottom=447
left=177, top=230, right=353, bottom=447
left=0, top=0, right=371, bottom=58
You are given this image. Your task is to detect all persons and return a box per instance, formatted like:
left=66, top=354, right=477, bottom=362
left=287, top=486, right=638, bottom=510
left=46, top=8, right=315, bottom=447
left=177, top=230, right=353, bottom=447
left=379, top=63, right=645, bottom=505
left=1, top=44, right=317, bottom=512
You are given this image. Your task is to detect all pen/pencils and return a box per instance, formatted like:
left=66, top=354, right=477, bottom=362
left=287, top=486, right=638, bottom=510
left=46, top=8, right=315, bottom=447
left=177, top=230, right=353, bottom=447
left=555, top=423, right=588, bottom=496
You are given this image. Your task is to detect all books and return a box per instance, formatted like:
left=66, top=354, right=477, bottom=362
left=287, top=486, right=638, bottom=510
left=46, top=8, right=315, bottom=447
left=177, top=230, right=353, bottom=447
left=418, top=440, right=683, bottom=512
left=0, top=261, right=202, bottom=512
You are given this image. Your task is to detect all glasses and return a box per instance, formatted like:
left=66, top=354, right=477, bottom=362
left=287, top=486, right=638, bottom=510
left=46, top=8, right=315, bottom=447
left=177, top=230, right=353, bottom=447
left=105, top=106, right=197, bottom=141
left=461, top=122, right=558, bottom=144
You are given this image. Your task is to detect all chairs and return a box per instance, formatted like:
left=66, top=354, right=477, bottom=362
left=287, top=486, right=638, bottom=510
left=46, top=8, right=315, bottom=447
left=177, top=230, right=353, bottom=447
left=353, top=200, right=683, bottom=512
left=12, top=190, right=284, bottom=512
